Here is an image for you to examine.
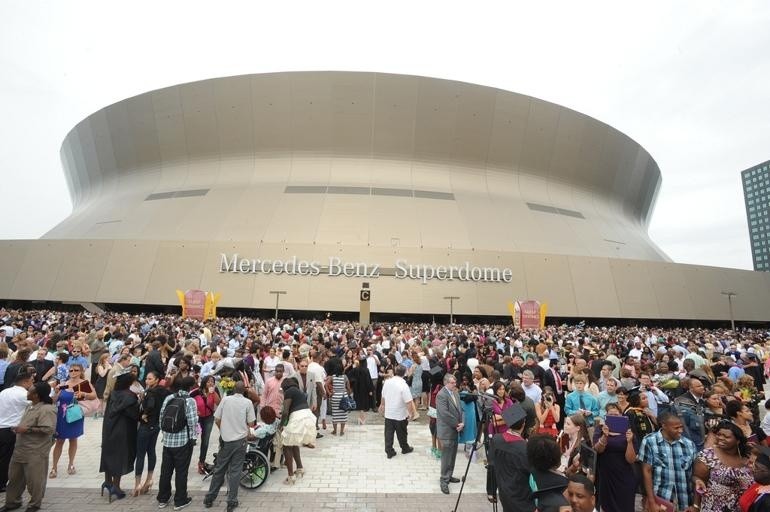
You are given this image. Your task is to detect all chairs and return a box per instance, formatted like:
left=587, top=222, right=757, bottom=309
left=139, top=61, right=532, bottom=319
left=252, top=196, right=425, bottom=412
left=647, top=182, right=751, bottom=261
left=173, top=496, right=193, bottom=511
left=66, top=463, right=78, bottom=475
left=49, top=466, right=57, bottom=479
left=303, top=431, right=345, bottom=449
left=386, top=450, right=397, bottom=459
left=156, top=496, right=172, bottom=508
left=401, top=446, right=414, bottom=454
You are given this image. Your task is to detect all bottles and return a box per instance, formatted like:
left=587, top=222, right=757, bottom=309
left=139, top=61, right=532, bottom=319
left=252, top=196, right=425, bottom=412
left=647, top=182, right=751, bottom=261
left=502, top=404, right=527, bottom=430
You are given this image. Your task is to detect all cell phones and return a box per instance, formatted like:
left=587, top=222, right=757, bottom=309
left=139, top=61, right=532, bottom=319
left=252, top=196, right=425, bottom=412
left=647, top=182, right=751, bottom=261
left=543, top=394, right=551, bottom=402
left=58, top=383, right=69, bottom=391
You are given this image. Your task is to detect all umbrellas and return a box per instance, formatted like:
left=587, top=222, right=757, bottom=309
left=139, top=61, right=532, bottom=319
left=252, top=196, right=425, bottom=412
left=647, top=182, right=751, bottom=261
left=225, top=501, right=239, bottom=512
left=449, top=476, right=460, bottom=484
left=205, top=500, right=213, bottom=508
left=25, top=505, right=40, bottom=512
left=439, top=482, right=451, bottom=495
left=0, top=502, right=21, bottom=512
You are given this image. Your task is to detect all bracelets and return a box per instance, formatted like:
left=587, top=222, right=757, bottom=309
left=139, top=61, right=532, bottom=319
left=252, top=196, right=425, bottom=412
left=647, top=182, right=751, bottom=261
left=598, top=439, right=608, bottom=446
left=83, top=392, right=86, bottom=400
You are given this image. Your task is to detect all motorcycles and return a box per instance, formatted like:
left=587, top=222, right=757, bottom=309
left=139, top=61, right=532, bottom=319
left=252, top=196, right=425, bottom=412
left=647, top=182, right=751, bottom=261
left=452, top=410, right=505, bottom=512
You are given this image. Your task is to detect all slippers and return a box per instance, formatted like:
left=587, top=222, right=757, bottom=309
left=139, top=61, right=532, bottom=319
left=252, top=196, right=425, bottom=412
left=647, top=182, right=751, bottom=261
left=160, top=392, right=193, bottom=433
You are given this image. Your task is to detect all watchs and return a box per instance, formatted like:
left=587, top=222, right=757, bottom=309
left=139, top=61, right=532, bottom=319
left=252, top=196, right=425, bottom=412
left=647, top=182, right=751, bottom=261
left=27, top=426, right=32, bottom=433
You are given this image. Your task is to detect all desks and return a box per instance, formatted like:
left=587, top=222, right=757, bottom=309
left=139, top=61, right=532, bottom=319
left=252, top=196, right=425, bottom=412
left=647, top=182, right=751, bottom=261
left=269, top=289, right=286, bottom=319
left=443, top=295, right=460, bottom=325
left=720, top=291, right=739, bottom=331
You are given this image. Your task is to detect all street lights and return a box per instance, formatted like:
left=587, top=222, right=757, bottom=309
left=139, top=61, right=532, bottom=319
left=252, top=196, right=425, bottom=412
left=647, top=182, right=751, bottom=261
left=339, top=395, right=357, bottom=412
left=65, top=402, right=83, bottom=424
left=77, top=395, right=101, bottom=416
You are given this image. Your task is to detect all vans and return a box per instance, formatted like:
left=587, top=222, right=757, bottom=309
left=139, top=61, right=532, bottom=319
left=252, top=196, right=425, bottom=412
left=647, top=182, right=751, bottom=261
left=107, top=483, right=126, bottom=501
left=141, top=480, right=153, bottom=496
left=284, top=475, right=297, bottom=486
left=100, top=481, right=111, bottom=497
left=129, top=483, right=141, bottom=496
left=293, top=468, right=305, bottom=478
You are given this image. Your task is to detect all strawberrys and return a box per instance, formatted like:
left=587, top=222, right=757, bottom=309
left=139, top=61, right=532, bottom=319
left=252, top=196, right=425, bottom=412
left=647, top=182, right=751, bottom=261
left=200, top=412, right=295, bottom=491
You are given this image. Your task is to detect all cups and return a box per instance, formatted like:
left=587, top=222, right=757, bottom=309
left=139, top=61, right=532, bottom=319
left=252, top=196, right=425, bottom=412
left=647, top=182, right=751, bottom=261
left=73, top=350, right=81, bottom=353
left=69, top=368, right=80, bottom=373
left=300, top=363, right=308, bottom=367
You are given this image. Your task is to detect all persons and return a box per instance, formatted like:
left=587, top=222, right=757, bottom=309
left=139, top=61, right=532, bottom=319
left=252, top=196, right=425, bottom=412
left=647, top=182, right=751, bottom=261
left=199, top=380, right=256, bottom=510
left=0, top=306, right=770, bottom=512
left=378, top=364, right=418, bottom=459
left=3, top=382, right=57, bottom=511
left=159, top=376, right=200, bottom=511
left=97, top=373, right=142, bottom=505
left=436, top=374, right=464, bottom=495
left=326, top=364, right=352, bottom=437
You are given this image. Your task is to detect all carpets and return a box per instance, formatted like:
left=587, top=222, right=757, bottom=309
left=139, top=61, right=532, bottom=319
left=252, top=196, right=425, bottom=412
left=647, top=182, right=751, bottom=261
left=579, top=394, right=586, bottom=413
left=555, top=373, right=562, bottom=392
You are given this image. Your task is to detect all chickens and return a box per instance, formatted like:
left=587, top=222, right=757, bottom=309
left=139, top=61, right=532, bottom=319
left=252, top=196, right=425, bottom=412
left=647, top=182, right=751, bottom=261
left=604, top=415, right=630, bottom=435
left=578, top=443, right=598, bottom=474
